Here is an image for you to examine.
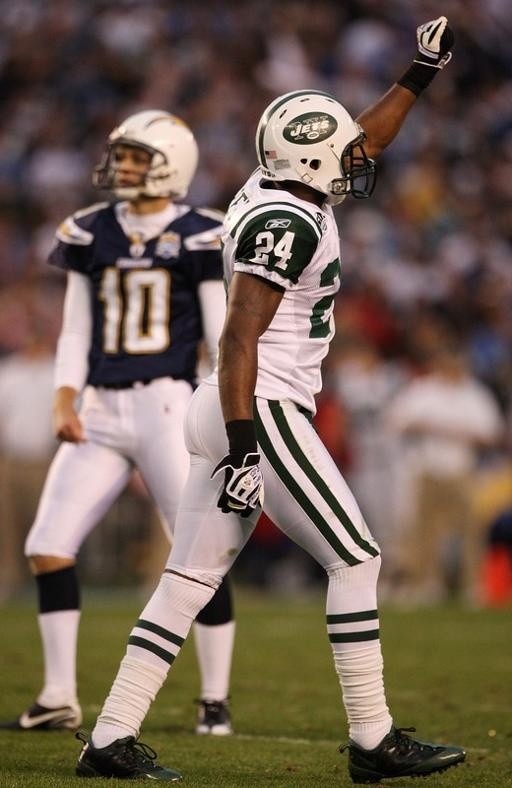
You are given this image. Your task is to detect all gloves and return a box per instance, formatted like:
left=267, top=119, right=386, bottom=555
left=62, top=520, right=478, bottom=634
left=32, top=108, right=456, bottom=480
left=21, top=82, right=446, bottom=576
left=414, top=15, right=454, bottom=69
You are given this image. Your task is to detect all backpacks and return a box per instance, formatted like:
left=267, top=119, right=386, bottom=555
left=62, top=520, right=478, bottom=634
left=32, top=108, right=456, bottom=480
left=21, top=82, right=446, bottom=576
left=213, top=451, right=267, bottom=516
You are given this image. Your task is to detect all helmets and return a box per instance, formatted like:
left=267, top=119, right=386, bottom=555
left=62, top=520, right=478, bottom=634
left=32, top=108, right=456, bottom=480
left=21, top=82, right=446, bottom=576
left=255, top=89, right=377, bottom=205
left=92, top=110, right=197, bottom=203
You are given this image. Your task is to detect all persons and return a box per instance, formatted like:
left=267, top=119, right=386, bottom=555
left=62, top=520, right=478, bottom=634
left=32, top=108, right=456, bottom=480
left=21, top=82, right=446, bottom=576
left=3, top=4, right=512, bottom=613
left=0, top=111, right=232, bottom=740
left=72, top=12, right=464, bottom=785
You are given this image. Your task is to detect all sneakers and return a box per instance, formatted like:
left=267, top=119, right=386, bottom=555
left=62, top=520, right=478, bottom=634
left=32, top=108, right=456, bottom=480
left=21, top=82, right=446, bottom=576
left=74, top=732, right=183, bottom=782
left=338, top=728, right=465, bottom=784
left=195, top=697, right=233, bottom=735
left=3, top=700, right=82, bottom=730
